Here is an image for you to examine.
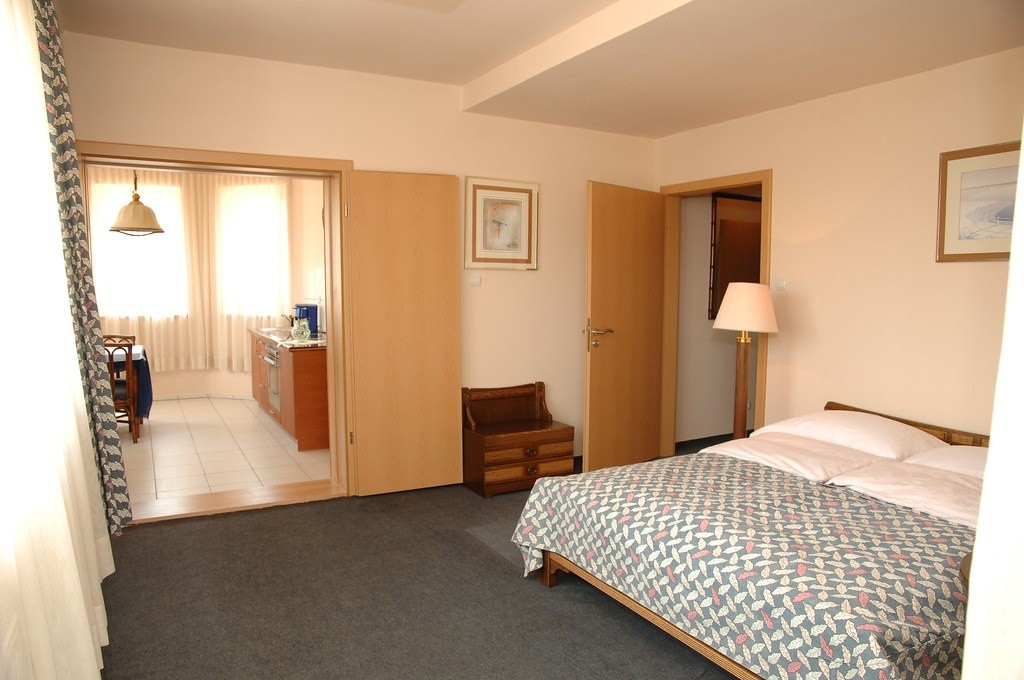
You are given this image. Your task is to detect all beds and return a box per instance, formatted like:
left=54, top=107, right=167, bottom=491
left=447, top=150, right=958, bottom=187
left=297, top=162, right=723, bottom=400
left=511, top=401, right=990, bottom=680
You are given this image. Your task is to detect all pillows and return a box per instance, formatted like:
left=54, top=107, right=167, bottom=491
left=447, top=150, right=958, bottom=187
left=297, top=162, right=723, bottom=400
left=902, top=445, right=988, bottom=480
left=749, top=409, right=951, bottom=461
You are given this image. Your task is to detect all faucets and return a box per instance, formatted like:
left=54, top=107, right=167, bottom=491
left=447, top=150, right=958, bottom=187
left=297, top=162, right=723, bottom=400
left=280, top=314, right=291, bottom=328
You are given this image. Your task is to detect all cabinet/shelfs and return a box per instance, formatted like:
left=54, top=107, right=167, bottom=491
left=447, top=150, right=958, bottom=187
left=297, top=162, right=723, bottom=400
left=249, top=327, right=329, bottom=453
left=462, top=382, right=574, bottom=499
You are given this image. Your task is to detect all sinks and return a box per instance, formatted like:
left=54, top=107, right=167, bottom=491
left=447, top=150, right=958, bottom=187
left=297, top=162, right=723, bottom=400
left=265, top=330, right=292, bottom=339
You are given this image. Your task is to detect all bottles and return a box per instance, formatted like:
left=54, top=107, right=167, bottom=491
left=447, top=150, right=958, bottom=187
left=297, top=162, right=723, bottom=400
left=292, top=317, right=311, bottom=341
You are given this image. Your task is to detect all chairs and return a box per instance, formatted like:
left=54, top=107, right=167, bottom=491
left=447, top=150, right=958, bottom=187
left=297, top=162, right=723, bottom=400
left=103, top=343, right=137, bottom=443
left=102, top=335, right=143, bottom=432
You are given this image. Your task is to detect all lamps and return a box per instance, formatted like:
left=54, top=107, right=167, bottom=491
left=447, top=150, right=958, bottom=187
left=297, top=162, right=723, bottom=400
left=712, top=281, right=780, bottom=440
left=109, top=169, right=164, bottom=236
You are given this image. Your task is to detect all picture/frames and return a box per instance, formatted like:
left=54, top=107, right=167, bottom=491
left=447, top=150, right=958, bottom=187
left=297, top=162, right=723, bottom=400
left=707, top=193, right=762, bottom=320
left=463, top=177, right=538, bottom=271
left=936, top=139, right=1022, bottom=262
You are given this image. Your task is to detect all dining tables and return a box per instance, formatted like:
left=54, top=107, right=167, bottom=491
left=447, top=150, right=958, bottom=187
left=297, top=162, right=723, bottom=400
left=105, top=346, right=153, bottom=438
